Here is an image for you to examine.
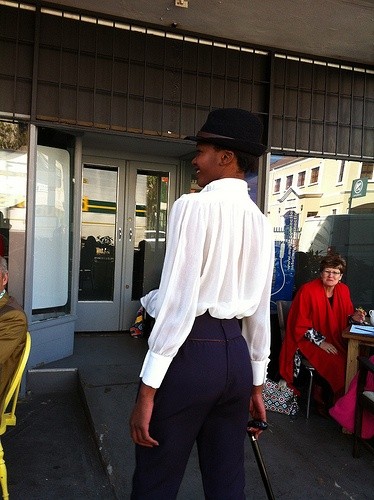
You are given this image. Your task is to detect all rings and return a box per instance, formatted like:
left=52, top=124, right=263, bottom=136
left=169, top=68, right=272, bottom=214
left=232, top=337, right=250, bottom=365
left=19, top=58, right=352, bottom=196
left=329, top=348, right=331, bottom=351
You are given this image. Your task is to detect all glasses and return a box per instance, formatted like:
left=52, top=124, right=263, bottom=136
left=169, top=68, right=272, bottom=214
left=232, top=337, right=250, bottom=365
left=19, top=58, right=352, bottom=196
left=322, top=271, right=342, bottom=276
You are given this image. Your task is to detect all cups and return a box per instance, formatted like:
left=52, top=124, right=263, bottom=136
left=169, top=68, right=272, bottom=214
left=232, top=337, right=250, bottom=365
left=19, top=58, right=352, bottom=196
left=369, top=310, right=374, bottom=326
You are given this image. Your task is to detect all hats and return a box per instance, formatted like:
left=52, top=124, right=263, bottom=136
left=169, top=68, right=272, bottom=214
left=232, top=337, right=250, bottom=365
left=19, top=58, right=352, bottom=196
left=184, top=108, right=269, bottom=157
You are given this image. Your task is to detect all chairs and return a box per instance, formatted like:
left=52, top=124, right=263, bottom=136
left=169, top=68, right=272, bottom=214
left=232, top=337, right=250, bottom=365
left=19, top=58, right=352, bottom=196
left=351, top=355, right=374, bottom=459
left=0, top=330, right=33, bottom=500
left=275, top=300, right=317, bottom=419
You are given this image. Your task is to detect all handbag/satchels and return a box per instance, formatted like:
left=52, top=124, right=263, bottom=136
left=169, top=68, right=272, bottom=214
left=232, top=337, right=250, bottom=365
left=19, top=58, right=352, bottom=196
left=261, top=378, right=301, bottom=417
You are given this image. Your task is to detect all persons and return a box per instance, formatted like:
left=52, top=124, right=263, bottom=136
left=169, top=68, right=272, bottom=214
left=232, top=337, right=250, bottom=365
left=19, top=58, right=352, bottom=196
left=0, top=256, right=28, bottom=426
left=279, top=255, right=367, bottom=419
left=129, top=108, right=272, bottom=500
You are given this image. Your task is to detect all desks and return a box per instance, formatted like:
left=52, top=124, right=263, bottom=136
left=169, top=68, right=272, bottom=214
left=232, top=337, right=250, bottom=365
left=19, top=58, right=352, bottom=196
left=341, top=324, right=374, bottom=434
